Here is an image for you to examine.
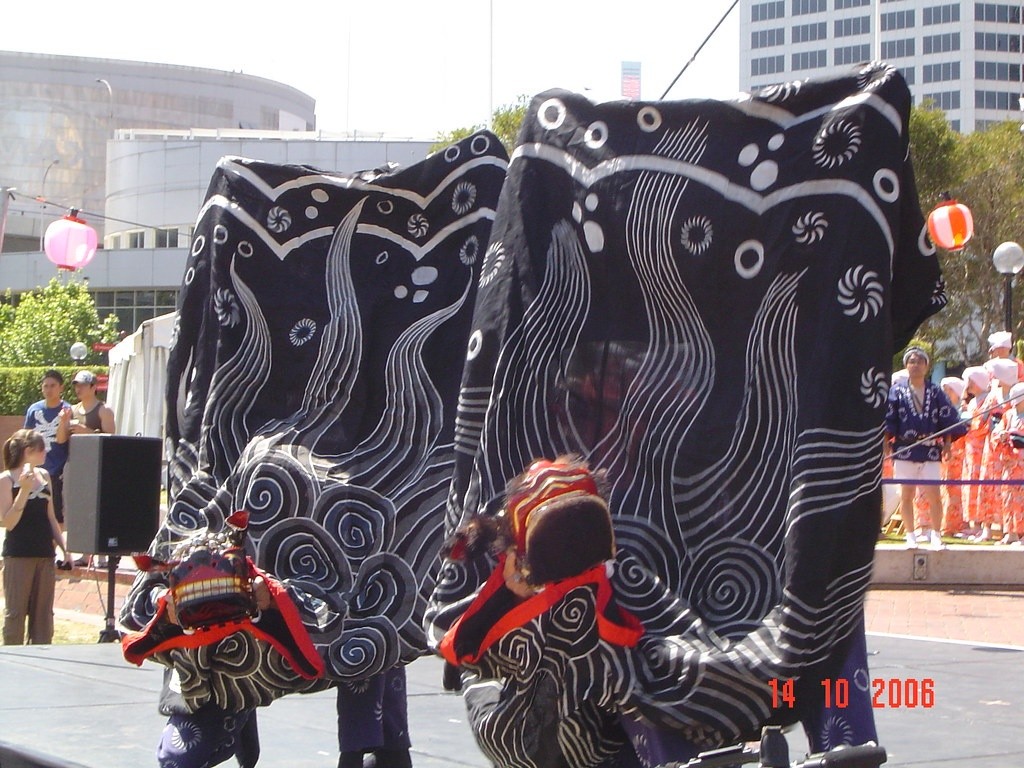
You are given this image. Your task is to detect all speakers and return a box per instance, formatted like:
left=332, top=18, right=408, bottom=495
left=64, top=432, right=164, bottom=556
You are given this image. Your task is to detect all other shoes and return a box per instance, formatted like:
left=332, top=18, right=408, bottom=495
left=98, top=560, right=108, bottom=568
left=73, top=558, right=92, bottom=566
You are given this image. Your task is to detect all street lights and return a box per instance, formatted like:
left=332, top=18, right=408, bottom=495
left=993, top=241, right=1024, bottom=355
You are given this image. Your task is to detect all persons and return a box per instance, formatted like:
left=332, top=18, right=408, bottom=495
left=56, top=369, right=114, bottom=567
left=884, top=331, right=1024, bottom=544
left=22, top=369, right=73, bottom=551
left=0, top=429, right=70, bottom=645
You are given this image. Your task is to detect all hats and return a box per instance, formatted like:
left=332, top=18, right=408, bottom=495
left=72, top=370, right=97, bottom=385
